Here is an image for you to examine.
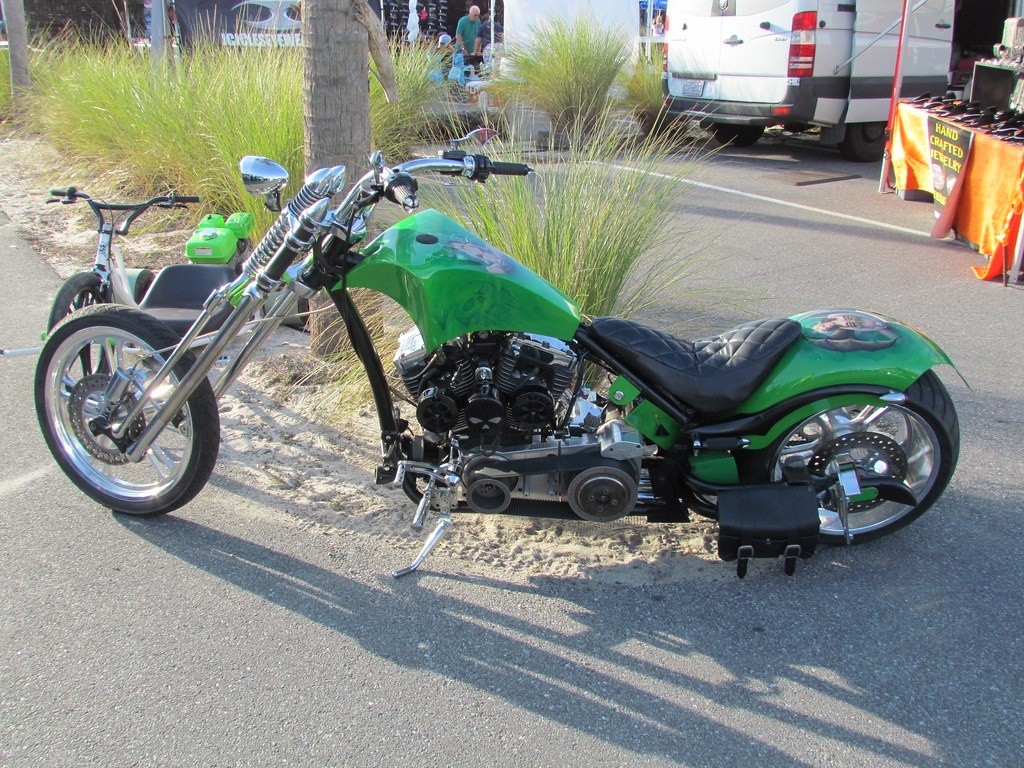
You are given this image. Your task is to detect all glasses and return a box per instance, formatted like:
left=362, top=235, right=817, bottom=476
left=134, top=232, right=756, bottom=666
left=470, top=13, right=481, bottom=17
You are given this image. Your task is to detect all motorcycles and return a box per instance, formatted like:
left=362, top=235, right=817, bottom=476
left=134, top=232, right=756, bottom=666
left=32, top=123, right=977, bottom=583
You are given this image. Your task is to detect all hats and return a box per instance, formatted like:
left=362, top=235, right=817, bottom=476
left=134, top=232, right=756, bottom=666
left=439, top=34, right=452, bottom=46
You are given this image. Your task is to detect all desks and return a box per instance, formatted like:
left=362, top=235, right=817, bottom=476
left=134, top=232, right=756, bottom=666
left=893, top=100, right=1024, bottom=281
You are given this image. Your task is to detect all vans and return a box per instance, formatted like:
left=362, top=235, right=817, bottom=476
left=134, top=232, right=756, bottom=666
left=463, top=0, right=689, bottom=139
left=660, top=0, right=1024, bottom=163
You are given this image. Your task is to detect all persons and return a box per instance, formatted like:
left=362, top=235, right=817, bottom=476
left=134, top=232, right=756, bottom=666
left=652, top=16, right=664, bottom=35
left=455, top=5, right=482, bottom=56
left=474, top=14, right=503, bottom=54
left=435, top=35, right=454, bottom=56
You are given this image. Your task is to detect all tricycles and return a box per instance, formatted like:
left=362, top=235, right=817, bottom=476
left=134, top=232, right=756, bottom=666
left=39, top=186, right=257, bottom=395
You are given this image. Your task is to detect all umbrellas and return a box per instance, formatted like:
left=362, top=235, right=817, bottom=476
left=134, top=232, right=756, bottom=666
left=639, top=0, right=668, bottom=16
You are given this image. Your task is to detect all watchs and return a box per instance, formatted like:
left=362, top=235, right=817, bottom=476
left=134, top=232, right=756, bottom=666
left=461, top=47, right=465, bottom=51
